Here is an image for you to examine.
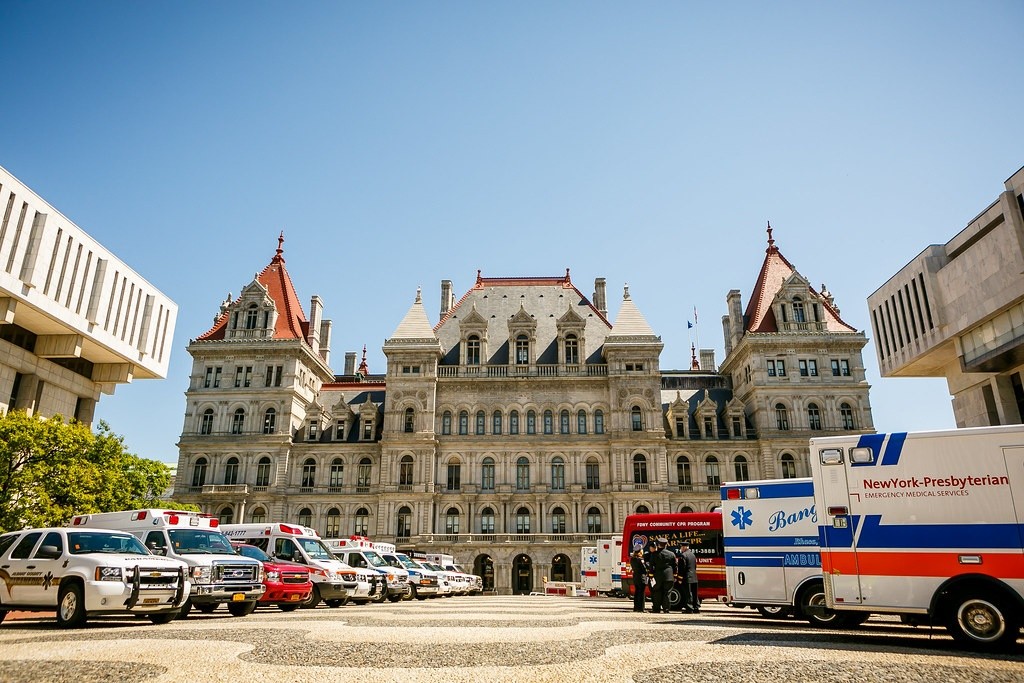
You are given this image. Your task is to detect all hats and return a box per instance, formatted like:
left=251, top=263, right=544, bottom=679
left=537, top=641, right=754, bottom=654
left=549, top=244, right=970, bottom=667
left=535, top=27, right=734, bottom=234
left=650, top=542, right=656, bottom=548
left=657, top=538, right=667, bottom=545
left=632, top=544, right=642, bottom=553
left=679, top=542, right=690, bottom=546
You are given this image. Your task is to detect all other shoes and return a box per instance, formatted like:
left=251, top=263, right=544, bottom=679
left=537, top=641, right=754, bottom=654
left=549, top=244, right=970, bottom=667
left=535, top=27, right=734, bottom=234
left=648, top=610, right=660, bottom=613
left=638, top=611, right=647, bottom=613
left=693, top=607, right=700, bottom=613
left=663, top=608, right=669, bottom=613
left=682, top=608, right=693, bottom=613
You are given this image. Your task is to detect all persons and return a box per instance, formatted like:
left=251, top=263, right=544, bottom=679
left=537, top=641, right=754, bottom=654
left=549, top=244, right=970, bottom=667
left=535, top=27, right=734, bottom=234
left=630, top=545, right=647, bottom=612
left=645, top=541, right=659, bottom=604
left=649, top=538, right=677, bottom=613
left=677, top=542, right=701, bottom=614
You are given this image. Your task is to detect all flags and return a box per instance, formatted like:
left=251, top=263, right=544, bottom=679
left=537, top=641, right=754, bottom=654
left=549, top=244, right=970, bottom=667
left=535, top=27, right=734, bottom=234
left=688, top=321, right=693, bottom=329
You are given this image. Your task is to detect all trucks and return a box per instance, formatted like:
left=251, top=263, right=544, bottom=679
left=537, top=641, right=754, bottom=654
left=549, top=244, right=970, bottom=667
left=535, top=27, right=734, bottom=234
left=597, top=535, right=635, bottom=600
left=810, top=424, right=1024, bottom=654
left=621, top=513, right=728, bottom=612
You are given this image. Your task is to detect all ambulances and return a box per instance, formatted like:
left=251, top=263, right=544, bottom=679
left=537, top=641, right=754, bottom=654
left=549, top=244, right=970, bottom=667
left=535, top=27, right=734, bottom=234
left=720, top=477, right=872, bottom=628
left=0, top=508, right=484, bottom=630
left=580, top=547, right=615, bottom=597
